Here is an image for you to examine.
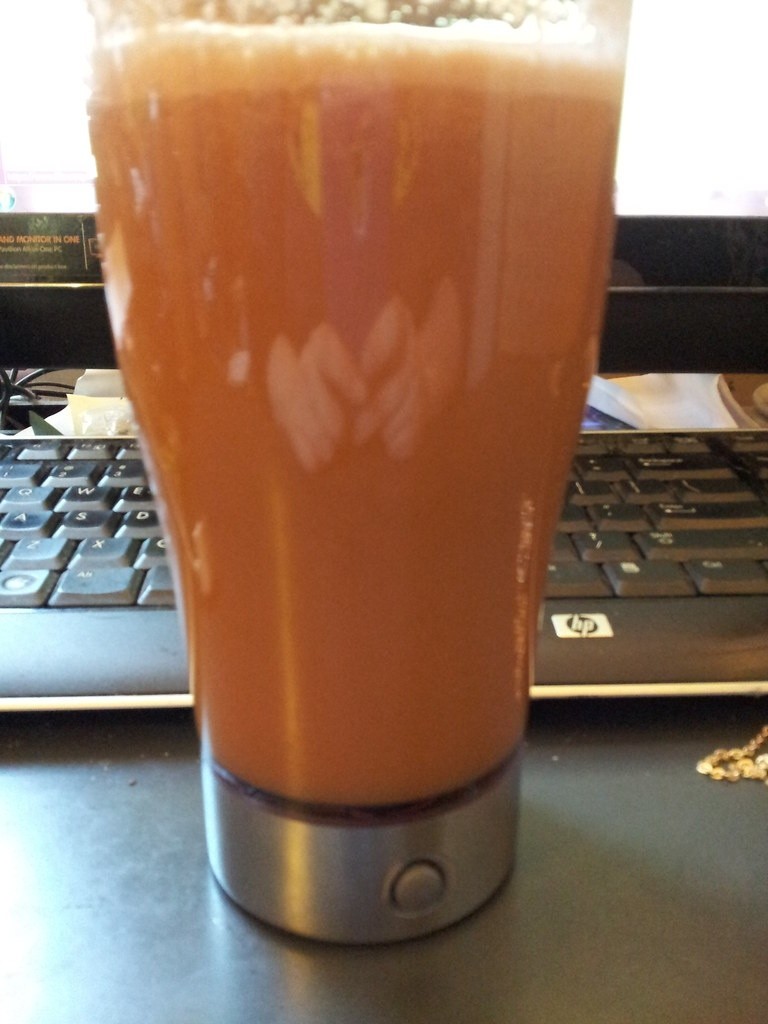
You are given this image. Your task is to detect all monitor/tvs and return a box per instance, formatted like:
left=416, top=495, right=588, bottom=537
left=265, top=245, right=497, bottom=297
left=0, top=0, right=768, bottom=375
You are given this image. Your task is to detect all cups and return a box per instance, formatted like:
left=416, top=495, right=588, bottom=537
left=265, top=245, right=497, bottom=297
left=76, top=0, right=637, bottom=946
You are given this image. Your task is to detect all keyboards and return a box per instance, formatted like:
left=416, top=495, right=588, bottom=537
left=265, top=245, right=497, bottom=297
left=0, top=428, right=768, bottom=712
